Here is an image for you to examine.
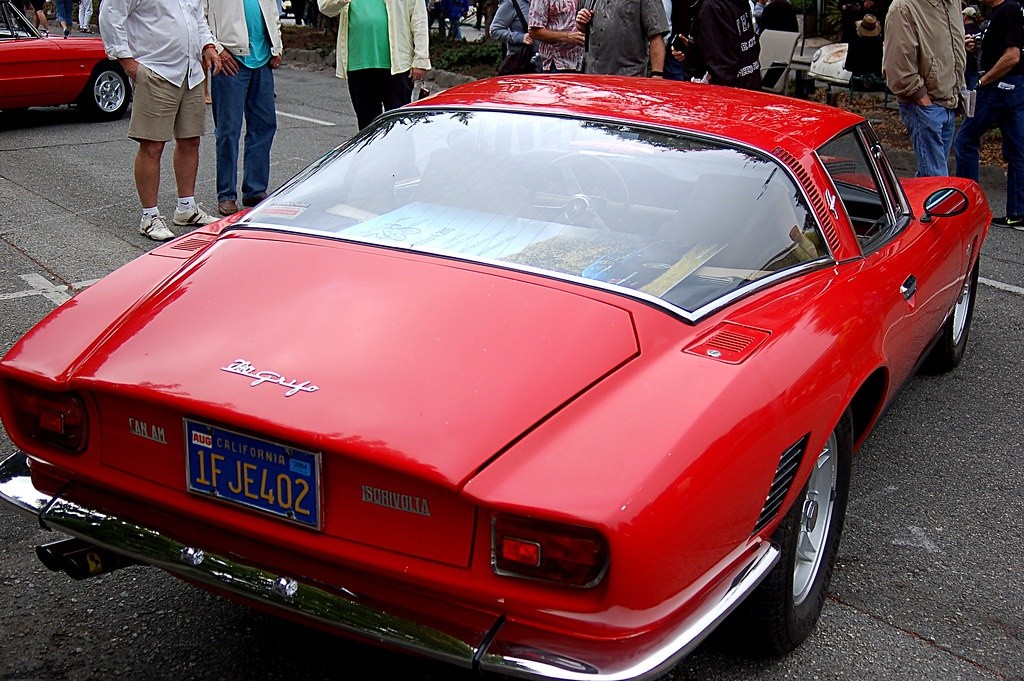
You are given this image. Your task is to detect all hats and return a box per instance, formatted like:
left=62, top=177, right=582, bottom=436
left=962, top=6, right=977, bottom=17
left=855, top=13, right=881, bottom=36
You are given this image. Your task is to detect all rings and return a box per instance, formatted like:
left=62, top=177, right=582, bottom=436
left=277, top=64, right=279, bottom=66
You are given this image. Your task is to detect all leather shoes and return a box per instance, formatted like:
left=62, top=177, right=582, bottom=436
left=219, top=199, right=238, bottom=215
left=242, top=194, right=269, bottom=207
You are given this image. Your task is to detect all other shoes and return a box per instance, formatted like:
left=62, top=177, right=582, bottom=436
left=63, top=28, right=70, bottom=38
left=473, top=23, right=481, bottom=29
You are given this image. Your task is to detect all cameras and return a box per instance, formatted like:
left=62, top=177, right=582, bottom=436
left=970, top=32, right=983, bottom=46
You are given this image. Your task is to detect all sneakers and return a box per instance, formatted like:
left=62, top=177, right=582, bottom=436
left=991, top=216, right=1024, bottom=230
left=138, top=214, right=175, bottom=241
left=173, top=201, right=221, bottom=225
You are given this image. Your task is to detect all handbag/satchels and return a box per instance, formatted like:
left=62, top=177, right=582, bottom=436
left=499, top=43, right=536, bottom=76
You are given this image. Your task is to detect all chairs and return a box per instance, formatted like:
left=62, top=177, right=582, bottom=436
left=660, top=174, right=798, bottom=267
left=759, top=29, right=801, bottom=97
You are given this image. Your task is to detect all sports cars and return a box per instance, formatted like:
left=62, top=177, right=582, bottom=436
left=0, top=0, right=133, bottom=122
left=0, top=73, right=993, bottom=681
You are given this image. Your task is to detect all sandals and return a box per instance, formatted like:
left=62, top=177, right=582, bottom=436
left=78, top=28, right=94, bottom=33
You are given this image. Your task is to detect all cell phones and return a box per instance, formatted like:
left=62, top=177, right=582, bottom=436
left=671, top=34, right=688, bottom=56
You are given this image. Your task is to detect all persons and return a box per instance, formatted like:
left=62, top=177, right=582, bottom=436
left=78, top=0, right=95, bottom=34
left=204, top=0, right=283, bottom=216
left=29, top=0, right=50, bottom=37
left=528, top=0, right=585, bottom=74
left=881, top=0, right=967, bottom=177
left=755, top=0, right=799, bottom=37
left=202, top=55, right=211, bottom=104
left=671, top=0, right=762, bottom=91
left=789, top=226, right=828, bottom=262
left=952, top=0, right=1024, bottom=230
left=962, top=7, right=983, bottom=91
left=317, top=0, right=432, bottom=131
left=56, top=0, right=73, bottom=39
left=98, top=0, right=222, bottom=241
left=489, top=0, right=541, bottom=75
left=575, top=0, right=670, bottom=79
left=845, top=14, right=883, bottom=78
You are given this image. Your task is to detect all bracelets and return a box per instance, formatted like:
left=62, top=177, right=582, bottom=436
left=205, top=45, right=215, bottom=49
left=651, top=71, right=663, bottom=76
